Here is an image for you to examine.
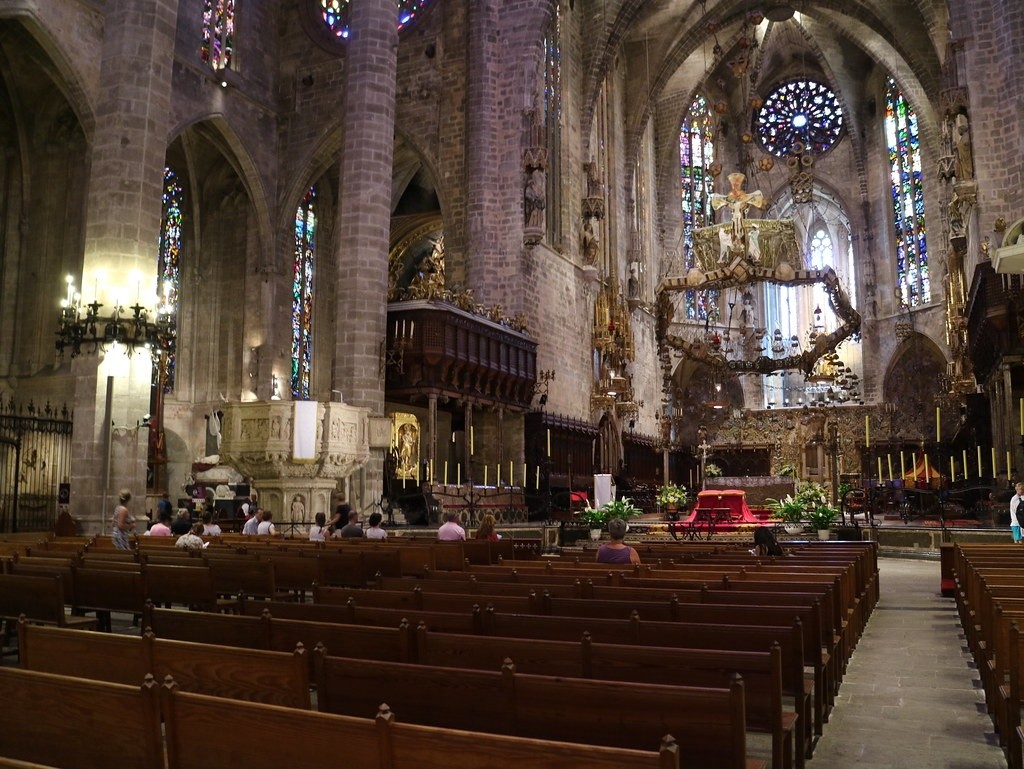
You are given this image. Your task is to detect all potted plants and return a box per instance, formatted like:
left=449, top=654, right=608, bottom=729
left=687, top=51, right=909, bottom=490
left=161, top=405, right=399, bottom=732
left=607, top=499, right=639, bottom=531
left=809, top=506, right=839, bottom=542
left=584, top=510, right=606, bottom=539
left=765, top=495, right=806, bottom=534
left=655, top=485, right=689, bottom=513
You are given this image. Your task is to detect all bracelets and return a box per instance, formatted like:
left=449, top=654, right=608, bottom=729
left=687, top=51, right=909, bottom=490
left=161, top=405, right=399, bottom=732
left=279, top=531, right=281, bottom=535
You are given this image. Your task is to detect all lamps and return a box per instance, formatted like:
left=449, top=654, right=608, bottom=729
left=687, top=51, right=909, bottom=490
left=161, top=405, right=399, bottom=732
left=56, top=271, right=157, bottom=354
left=534, top=359, right=556, bottom=392
left=380, top=318, right=415, bottom=373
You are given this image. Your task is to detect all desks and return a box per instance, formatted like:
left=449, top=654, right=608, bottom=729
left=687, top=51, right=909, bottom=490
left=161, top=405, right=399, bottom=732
left=695, top=508, right=733, bottom=525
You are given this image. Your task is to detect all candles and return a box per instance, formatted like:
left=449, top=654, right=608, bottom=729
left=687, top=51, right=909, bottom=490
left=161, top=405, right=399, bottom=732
left=402, top=459, right=462, bottom=488
left=888, top=454, right=892, bottom=480
left=937, top=406, right=941, bottom=442
left=877, top=457, right=882, bottom=483
left=523, top=463, right=526, bottom=486
left=977, top=446, right=982, bottom=476
left=1007, top=451, right=1012, bottom=480
left=912, top=453, right=917, bottom=481
left=962, top=450, right=968, bottom=479
left=470, top=426, right=474, bottom=455
left=951, top=456, right=955, bottom=481
left=536, top=466, right=540, bottom=489
left=484, top=461, right=514, bottom=487
left=865, top=416, right=870, bottom=446
left=1020, top=398, right=1024, bottom=435
left=991, top=448, right=996, bottom=478
left=547, top=428, right=550, bottom=457
left=900, top=451, right=905, bottom=479
left=924, top=454, right=930, bottom=482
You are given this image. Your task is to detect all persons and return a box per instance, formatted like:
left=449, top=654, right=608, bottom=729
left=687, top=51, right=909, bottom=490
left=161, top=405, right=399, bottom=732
left=309, top=493, right=388, bottom=542
left=475, top=515, right=498, bottom=539
left=985, top=236, right=992, bottom=258
left=292, top=496, right=305, bottom=527
left=716, top=196, right=761, bottom=264
left=630, top=269, right=637, bottom=297
left=748, top=526, right=783, bottom=557
left=951, top=125, right=974, bottom=180
left=110, top=488, right=136, bottom=549
left=1010, top=482, right=1024, bottom=543
left=438, top=510, right=466, bottom=540
left=744, top=300, right=754, bottom=327
left=144, top=492, right=284, bottom=536
left=585, top=224, right=597, bottom=264
left=526, top=179, right=545, bottom=227
left=175, top=523, right=205, bottom=549
left=401, top=429, right=412, bottom=467
left=947, top=193, right=964, bottom=236
left=596, top=518, right=641, bottom=564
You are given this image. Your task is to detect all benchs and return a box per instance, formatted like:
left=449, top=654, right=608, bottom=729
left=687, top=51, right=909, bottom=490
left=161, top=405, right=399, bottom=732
left=0, top=529, right=1024, bottom=769
